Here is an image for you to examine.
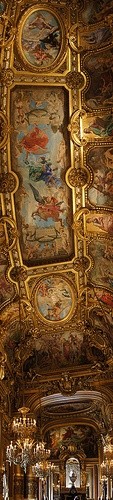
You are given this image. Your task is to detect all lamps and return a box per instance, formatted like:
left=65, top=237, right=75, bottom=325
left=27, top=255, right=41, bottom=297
left=54, top=371, right=80, bottom=397
left=99, top=434, right=113, bottom=473
left=29, top=460, right=55, bottom=484
left=8, top=387, right=49, bottom=474
